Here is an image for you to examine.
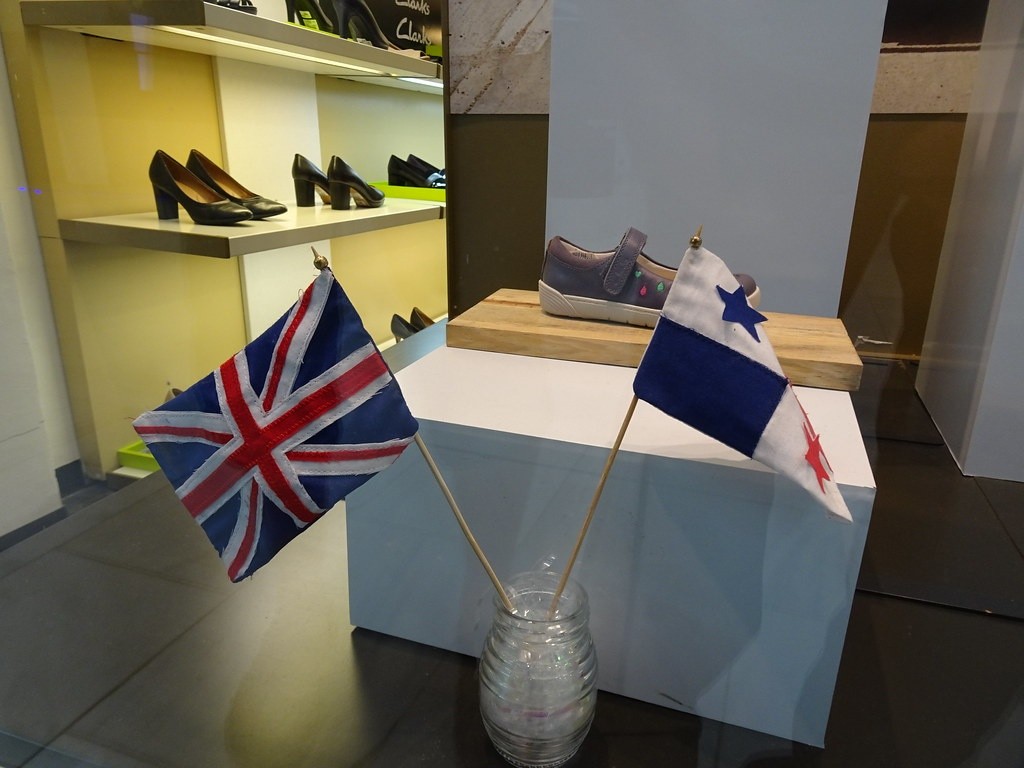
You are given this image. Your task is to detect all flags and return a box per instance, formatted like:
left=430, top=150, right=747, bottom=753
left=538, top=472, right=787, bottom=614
left=129, top=266, right=425, bottom=584
left=633, top=236, right=853, bottom=526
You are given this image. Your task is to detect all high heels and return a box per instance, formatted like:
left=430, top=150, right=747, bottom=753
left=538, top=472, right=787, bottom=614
left=390, top=307, right=436, bottom=344
left=292, top=154, right=386, bottom=210
left=286, top=0, right=430, bottom=61
left=149, top=148, right=289, bottom=225
left=388, top=153, right=445, bottom=188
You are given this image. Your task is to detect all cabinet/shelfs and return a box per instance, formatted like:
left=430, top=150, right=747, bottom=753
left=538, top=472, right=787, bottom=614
left=18, top=0, right=446, bottom=490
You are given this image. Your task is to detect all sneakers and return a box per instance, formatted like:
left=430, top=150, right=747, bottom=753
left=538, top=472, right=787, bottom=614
left=538, top=225, right=761, bottom=332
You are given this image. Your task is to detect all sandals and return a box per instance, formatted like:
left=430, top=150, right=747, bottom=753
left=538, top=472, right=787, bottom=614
left=203, top=0, right=258, bottom=15
